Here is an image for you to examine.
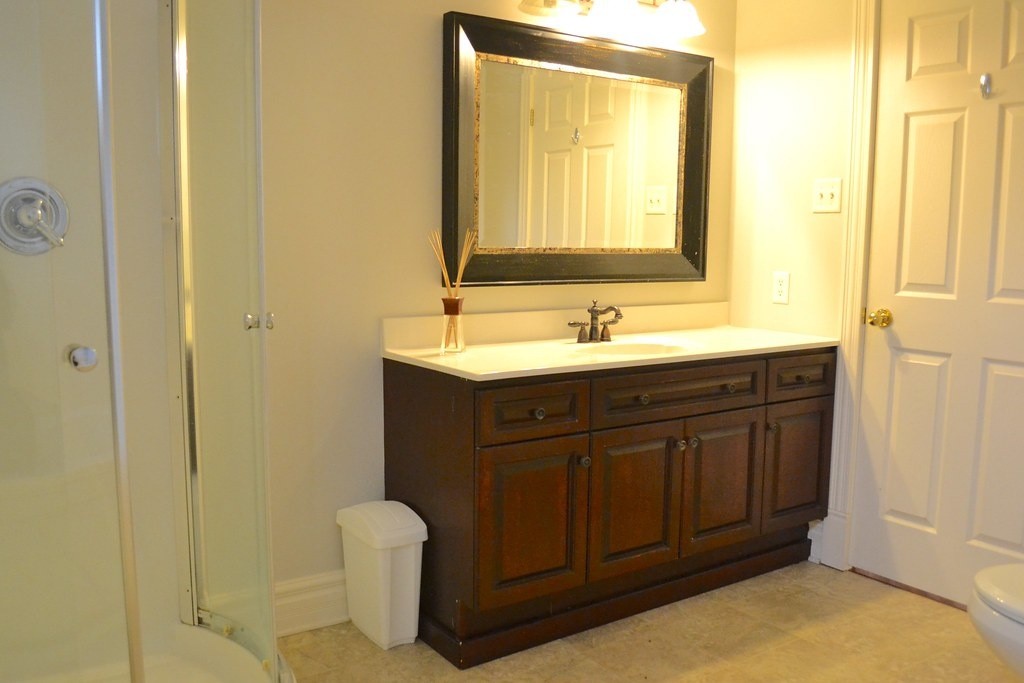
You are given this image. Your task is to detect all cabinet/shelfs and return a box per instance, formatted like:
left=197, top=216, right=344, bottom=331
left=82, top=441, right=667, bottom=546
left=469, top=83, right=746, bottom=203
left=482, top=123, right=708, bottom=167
left=382, top=347, right=836, bottom=671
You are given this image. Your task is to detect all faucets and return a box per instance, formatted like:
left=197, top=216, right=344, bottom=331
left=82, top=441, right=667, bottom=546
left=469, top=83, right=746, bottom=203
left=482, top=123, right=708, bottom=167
left=568, top=303, right=623, bottom=343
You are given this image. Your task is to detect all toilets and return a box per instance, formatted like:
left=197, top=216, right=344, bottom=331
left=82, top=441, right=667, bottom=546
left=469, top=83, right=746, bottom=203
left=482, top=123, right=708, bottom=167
left=967, top=561, right=1023, bottom=674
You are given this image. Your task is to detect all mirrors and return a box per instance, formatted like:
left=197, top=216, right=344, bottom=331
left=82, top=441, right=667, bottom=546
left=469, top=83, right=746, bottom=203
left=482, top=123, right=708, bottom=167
left=441, top=11, right=713, bottom=287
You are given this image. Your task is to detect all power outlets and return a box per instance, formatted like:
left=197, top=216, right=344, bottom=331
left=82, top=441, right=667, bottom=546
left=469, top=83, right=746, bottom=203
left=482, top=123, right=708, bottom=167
left=770, top=269, right=792, bottom=305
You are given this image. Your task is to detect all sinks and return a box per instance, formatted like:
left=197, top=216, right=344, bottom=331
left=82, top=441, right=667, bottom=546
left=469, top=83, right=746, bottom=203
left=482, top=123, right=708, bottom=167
left=564, top=341, right=679, bottom=360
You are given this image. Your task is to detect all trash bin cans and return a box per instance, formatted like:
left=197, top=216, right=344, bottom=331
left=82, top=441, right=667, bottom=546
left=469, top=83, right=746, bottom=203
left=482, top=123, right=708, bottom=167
left=338, top=498, right=430, bottom=645
